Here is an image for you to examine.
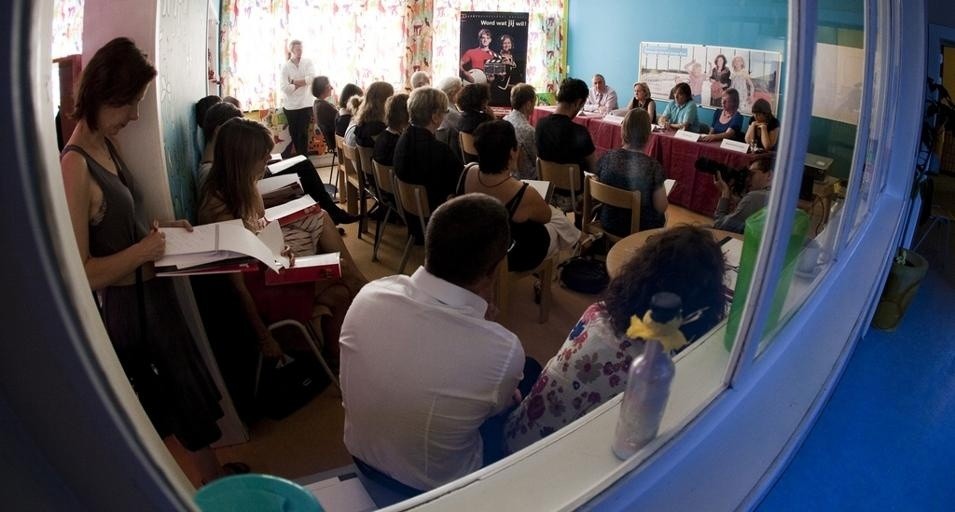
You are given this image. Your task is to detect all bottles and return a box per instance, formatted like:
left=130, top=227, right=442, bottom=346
left=610, top=292, right=687, bottom=464
left=819, top=193, right=848, bottom=269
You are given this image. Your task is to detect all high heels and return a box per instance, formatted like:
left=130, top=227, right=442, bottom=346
left=578, top=230, right=604, bottom=249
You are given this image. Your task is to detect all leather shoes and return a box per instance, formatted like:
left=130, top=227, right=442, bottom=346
left=329, top=208, right=370, bottom=226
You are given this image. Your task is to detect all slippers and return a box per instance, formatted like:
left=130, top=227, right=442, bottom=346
left=200, top=460, right=251, bottom=487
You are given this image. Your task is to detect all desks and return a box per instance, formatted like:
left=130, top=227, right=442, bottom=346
left=527, top=102, right=779, bottom=224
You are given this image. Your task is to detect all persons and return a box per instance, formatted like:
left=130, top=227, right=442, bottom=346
left=502, top=222, right=728, bottom=455
left=339, top=191, right=527, bottom=490
left=699, top=87, right=744, bottom=146
left=308, top=70, right=598, bottom=206
left=582, top=74, right=618, bottom=115
left=712, top=147, right=777, bottom=236
left=195, top=92, right=368, bottom=321
left=455, top=119, right=588, bottom=300
left=282, top=39, right=314, bottom=156
left=628, top=83, right=656, bottom=124
left=684, top=55, right=755, bottom=114
left=594, top=107, right=669, bottom=238
left=63, top=34, right=194, bottom=456
left=745, top=98, right=781, bottom=150
left=657, top=83, right=699, bottom=133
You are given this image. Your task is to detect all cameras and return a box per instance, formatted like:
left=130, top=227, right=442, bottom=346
left=694, top=157, right=752, bottom=197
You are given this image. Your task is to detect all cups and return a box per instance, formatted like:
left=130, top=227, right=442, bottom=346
left=797, top=238, right=831, bottom=272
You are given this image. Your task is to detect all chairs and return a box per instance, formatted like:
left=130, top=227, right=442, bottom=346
left=342, top=141, right=362, bottom=235
left=491, top=253, right=557, bottom=327
left=587, top=174, right=641, bottom=243
left=534, top=156, right=591, bottom=227
left=334, top=130, right=350, bottom=195
left=459, top=132, right=477, bottom=167
left=372, top=156, right=398, bottom=261
left=394, top=176, right=432, bottom=275
left=354, top=143, right=381, bottom=239
left=313, top=122, right=339, bottom=184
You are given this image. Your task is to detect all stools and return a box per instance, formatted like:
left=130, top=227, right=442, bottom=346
left=910, top=201, right=955, bottom=277
left=238, top=275, right=342, bottom=401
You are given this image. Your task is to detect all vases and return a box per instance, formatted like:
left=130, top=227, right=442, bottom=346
left=869, top=244, right=930, bottom=334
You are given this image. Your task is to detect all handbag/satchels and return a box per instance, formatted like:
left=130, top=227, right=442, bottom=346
left=556, top=256, right=610, bottom=295
left=252, top=349, right=335, bottom=420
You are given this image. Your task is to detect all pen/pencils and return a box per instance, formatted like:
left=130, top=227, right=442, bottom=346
left=153, top=219, right=159, bottom=233
left=723, top=249, right=730, bottom=255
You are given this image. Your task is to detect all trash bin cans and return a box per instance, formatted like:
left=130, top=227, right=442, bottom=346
left=873, top=252, right=929, bottom=330
left=194, top=473, right=326, bottom=511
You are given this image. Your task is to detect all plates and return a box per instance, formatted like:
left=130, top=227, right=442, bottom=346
left=794, top=263, right=824, bottom=280
